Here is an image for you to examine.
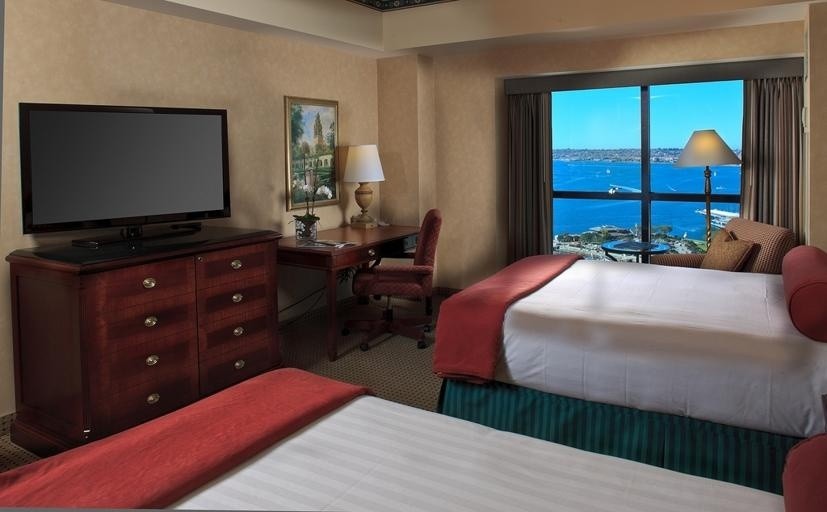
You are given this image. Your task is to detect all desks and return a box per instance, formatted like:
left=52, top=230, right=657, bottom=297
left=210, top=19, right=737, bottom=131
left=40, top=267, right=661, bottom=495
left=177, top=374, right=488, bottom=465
left=600, top=240, right=670, bottom=263
left=278, top=224, right=421, bottom=361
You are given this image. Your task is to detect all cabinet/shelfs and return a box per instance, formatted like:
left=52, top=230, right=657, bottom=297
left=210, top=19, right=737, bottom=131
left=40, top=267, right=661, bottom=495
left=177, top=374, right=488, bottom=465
left=3, top=226, right=285, bottom=458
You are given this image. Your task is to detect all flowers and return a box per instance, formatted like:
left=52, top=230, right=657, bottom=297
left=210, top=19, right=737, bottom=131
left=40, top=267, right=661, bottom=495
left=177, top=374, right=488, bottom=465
left=287, top=154, right=333, bottom=236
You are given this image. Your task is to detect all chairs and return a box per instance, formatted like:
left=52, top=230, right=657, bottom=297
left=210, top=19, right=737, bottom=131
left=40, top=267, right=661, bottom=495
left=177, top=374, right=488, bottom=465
left=649, top=218, right=795, bottom=275
left=341, top=207, right=442, bottom=351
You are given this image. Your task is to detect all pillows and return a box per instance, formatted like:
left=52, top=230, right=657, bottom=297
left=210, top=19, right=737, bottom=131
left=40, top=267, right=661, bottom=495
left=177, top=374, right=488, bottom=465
left=781, top=246, right=826, bottom=342
left=698, top=229, right=756, bottom=270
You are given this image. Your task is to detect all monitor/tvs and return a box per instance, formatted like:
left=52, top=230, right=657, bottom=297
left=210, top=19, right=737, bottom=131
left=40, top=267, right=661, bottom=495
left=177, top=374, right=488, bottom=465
left=18, top=101, right=232, bottom=250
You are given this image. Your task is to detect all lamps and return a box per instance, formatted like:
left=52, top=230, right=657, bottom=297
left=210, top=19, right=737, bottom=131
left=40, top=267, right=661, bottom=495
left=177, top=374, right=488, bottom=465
left=343, top=145, right=387, bottom=228
left=673, top=130, right=742, bottom=252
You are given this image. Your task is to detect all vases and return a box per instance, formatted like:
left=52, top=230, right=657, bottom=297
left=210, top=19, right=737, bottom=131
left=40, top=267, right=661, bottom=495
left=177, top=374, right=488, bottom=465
left=295, top=221, right=317, bottom=241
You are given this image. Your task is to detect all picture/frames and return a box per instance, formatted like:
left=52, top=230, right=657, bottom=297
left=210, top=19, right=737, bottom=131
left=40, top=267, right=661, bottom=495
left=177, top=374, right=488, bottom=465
left=283, top=95, right=340, bottom=213
